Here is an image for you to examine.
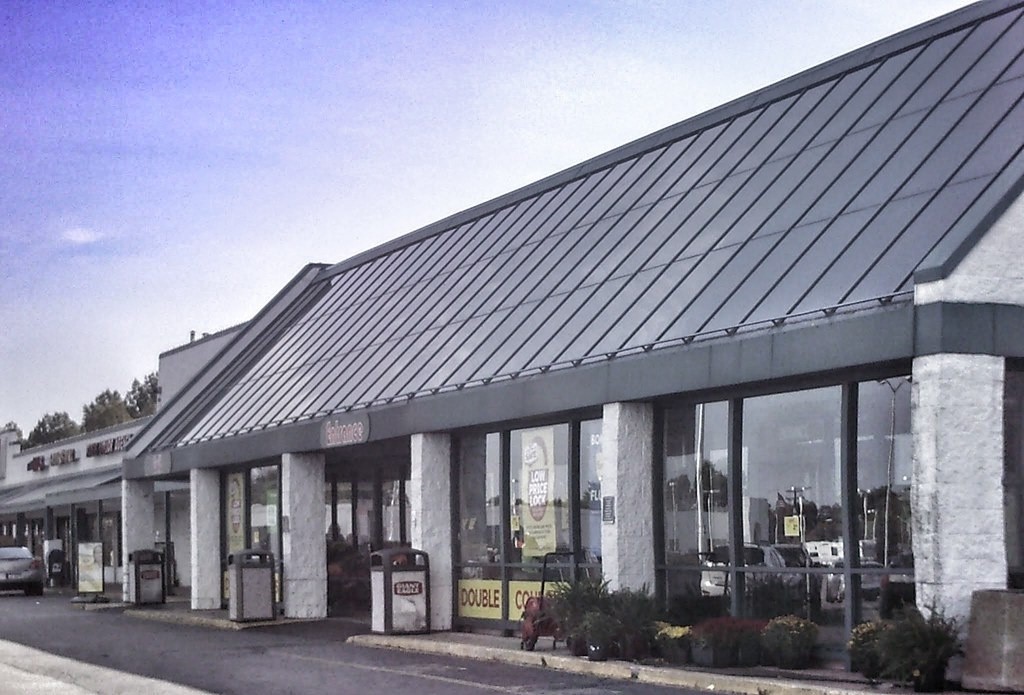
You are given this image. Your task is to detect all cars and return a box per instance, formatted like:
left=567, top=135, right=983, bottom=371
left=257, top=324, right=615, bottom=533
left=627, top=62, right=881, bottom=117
left=826, top=552, right=915, bottom=604
left=0, top=546, right=47, bottom=595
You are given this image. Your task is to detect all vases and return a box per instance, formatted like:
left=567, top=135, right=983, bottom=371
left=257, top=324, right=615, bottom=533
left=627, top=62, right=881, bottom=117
left=739, top=644, right=759, bottom=667
left=850, top=648, right=879, bottom=672
left=761, top=647, right=775, bottom=666
left=689, top=648, right=736, bottom=666
left=777, top=645, right=811, bottom=669
left=658, top=644, right=691, bottom=665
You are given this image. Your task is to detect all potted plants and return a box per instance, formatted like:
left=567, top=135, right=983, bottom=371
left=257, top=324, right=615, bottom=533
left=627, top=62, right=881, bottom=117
left=552, top=581, right=643, bottom=661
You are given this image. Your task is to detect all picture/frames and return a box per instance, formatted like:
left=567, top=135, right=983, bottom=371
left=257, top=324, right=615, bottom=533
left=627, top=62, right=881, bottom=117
left=911, top=658, right=949, bottom=692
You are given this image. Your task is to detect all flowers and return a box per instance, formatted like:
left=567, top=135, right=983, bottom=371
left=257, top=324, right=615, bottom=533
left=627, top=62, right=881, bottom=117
left=646, top=603, right=964, bottom=656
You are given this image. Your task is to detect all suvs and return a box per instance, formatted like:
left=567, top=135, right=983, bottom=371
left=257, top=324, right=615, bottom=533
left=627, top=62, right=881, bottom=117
left=699, top=542, right=824, bottom=613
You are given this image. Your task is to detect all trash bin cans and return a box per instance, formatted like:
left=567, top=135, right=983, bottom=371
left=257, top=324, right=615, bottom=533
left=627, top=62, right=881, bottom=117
left=128, top=549, right=166, bottom=606
left=370, top=548, right=431, bottom=636
left=227, top=549, right=276, bottom=622
